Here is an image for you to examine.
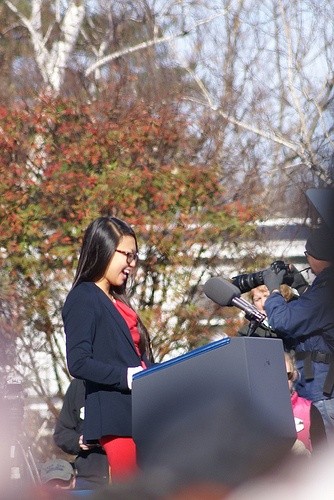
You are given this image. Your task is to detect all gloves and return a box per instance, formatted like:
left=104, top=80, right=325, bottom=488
left=263, top=266, right=286, bottom=293
left=286, top=264, right=308, bottom=295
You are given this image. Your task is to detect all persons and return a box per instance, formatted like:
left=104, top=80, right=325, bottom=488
left=236, top=228, right=334, bottom=461
left=62, top=216, right=160, bottom=484
left=40, top=376, right=111, bottom=489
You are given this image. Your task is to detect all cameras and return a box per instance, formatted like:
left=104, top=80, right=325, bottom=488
left=232, top=260, right=295, bottom=294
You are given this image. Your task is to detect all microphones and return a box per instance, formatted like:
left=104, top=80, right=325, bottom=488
left=205, top=277, right=274, bottom=331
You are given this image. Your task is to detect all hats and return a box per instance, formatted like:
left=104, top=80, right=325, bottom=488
left=41, top=458, right=74, bottom=485
left=306, top=229, right=334, bottom=261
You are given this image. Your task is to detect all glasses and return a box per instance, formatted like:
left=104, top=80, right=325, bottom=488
left=286, top=372, right=295, bottom=380
left=115, top=249, right=138, bottom=264
left=304, top=251, right=310, bottom=258
